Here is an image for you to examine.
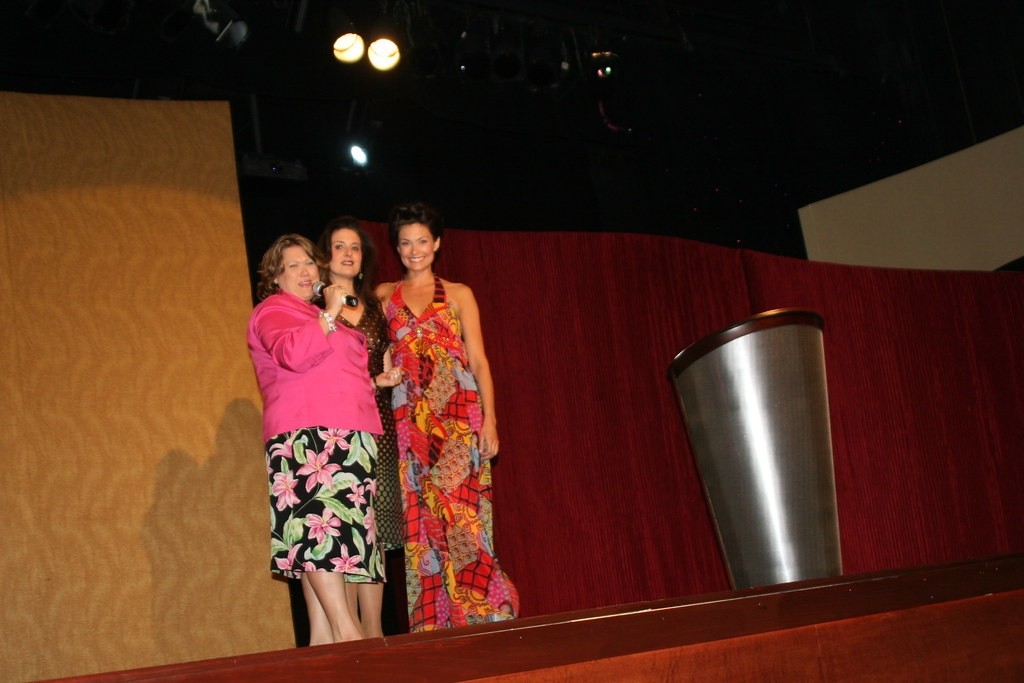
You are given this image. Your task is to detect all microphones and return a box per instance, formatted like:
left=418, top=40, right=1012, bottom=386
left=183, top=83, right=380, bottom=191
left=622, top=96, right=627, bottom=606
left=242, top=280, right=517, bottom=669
left=312, top=280, right=358, bottom=307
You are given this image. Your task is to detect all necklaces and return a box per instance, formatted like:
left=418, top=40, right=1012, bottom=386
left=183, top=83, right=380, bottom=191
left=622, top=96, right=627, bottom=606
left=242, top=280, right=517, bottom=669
left=342, top=293, right=360, bottom=310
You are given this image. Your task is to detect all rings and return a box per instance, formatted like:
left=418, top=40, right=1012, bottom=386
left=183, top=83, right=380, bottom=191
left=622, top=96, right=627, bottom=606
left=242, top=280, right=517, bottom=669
left=398, top=374, right=400, bottom=375
left=338, top=285, right=342, bottom=289
left=395, top=375, right=398, bottom=377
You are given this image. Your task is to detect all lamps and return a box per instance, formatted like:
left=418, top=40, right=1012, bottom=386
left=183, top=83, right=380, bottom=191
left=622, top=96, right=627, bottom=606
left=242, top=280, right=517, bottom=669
left=327, top=8, right=366, bottom=64
left=367, top=15, right=405, bottom=72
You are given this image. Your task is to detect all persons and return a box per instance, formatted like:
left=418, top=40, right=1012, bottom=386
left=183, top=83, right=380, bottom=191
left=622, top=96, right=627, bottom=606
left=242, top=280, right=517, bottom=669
left=247, top=216, right=405, bottom=646
left=375, top=204, right=519, bottom=632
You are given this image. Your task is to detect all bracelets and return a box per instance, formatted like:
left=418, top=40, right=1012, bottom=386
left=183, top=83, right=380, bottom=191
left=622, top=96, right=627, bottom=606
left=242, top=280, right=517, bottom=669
left=318, top=311, right=337, bottom=334
